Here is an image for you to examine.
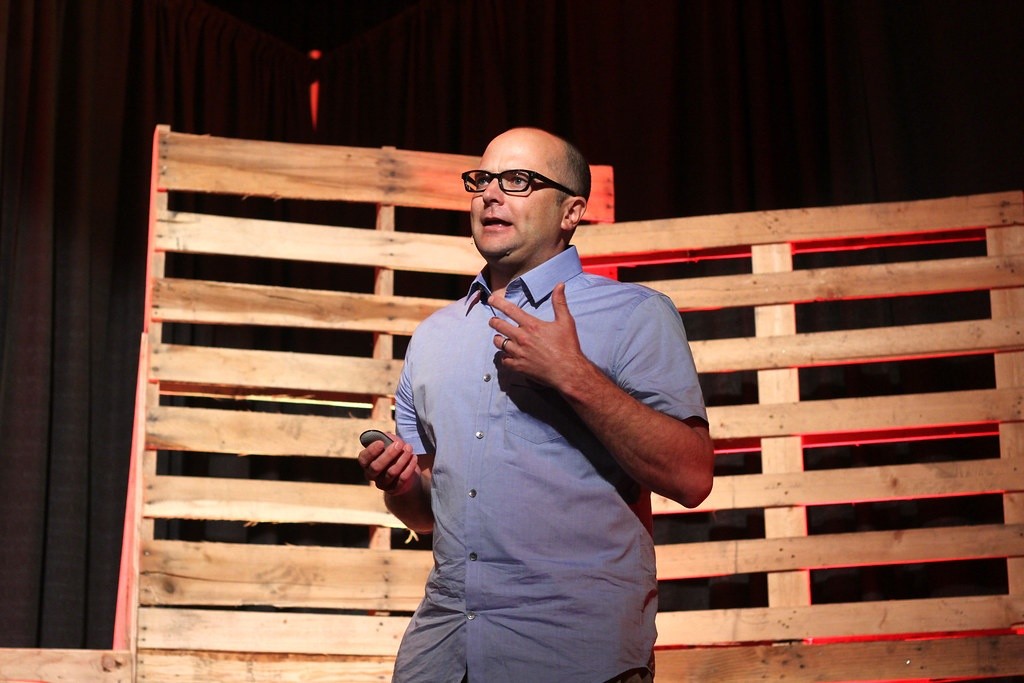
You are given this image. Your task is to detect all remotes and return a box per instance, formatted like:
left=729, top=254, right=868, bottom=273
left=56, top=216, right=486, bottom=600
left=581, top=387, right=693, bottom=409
left=360, top=430, right=394, bottom=452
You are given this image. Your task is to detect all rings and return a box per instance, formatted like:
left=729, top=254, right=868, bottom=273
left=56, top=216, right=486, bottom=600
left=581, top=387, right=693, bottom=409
left=502, top=339, right=509, bottom=350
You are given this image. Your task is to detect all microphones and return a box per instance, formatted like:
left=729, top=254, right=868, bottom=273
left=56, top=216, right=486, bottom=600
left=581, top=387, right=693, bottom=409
left=470, top=236, right=474, bottom=244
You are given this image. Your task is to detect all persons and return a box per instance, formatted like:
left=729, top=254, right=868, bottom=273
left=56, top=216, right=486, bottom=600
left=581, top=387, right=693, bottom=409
left=360, top=127, right=714, bottom=683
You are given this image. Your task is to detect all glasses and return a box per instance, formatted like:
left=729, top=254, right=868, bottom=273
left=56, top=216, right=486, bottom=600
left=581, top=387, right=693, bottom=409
left=462, top=167, right=576, bottom=196
